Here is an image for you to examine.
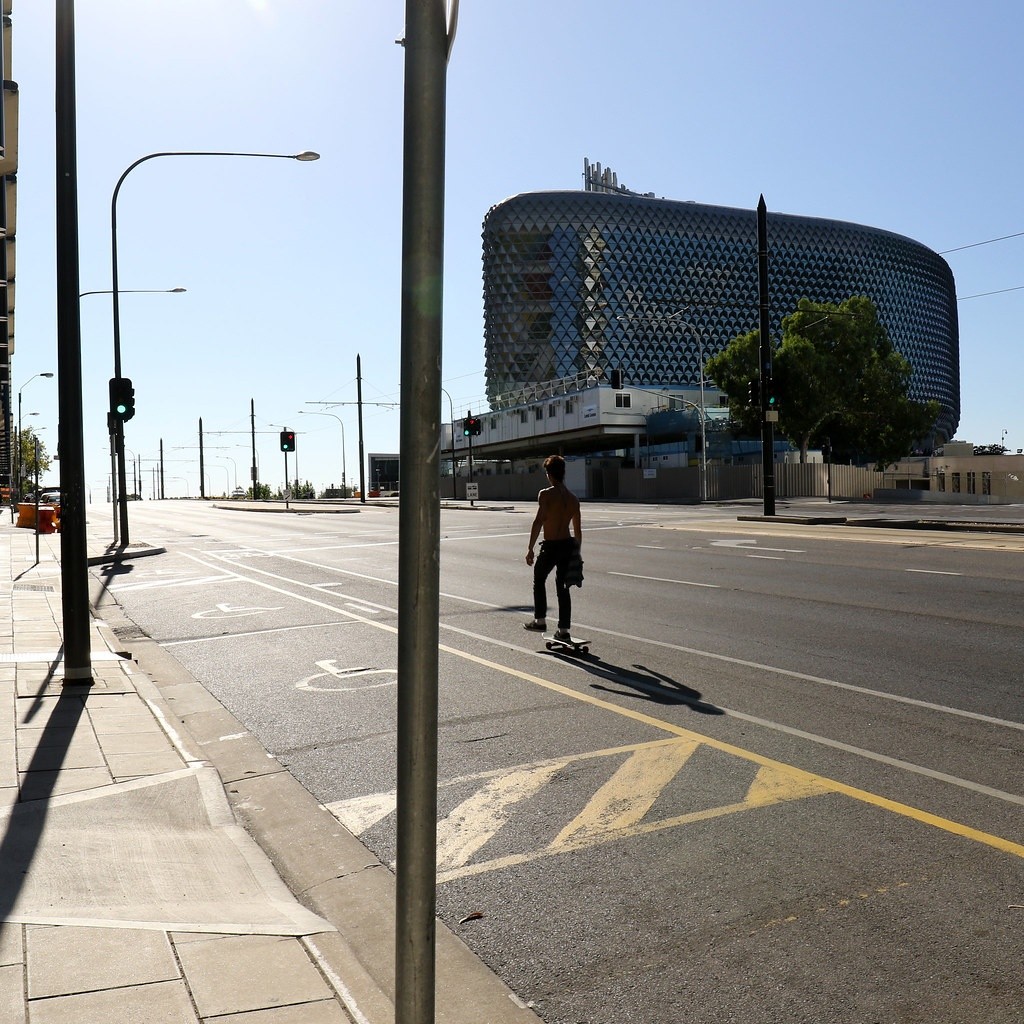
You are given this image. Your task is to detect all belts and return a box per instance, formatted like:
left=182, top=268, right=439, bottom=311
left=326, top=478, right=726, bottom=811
left=539, top=541, right=569, bottom=545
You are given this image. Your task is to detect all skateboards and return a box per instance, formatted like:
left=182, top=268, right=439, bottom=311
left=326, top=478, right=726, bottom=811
left=541, top=631, right=593, bottom=656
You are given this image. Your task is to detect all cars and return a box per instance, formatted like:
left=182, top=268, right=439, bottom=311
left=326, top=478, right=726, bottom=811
left=45, top=495, right=61, bottom=516
left=231, top=489, right=246, bottom=499
left=33, top=489, right=42, bottom=495
left=38, top=492, right=60, bottom=503
left=38, top=486, right=60, bottom=502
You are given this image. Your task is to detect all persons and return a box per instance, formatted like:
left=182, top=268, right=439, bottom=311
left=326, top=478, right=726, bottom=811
left=524, top=455, right=582, bottom=641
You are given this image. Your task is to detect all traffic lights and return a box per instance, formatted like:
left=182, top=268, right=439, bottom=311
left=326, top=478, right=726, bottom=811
left=463, top=418, right=481, bottom=435
left=109, top=378, right=135, bottom=422
left=280, top=432, right=295, bottom=451
left=764, top=377, right=783, bottom=407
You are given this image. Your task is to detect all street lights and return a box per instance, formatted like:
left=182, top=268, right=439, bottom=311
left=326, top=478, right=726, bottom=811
left=298, top=411, right=346, bottom=499
left=14, top=413, right=40, bottom=503
left=54, top=288, right=187, bottom=519
left=18, top=373, right=54, bottom=503
left=216, top=456, right=236, bottom=488
left=112, top=152, right=321, bottom=544
left=269, top=424, right=298, bottom=499
left=236, top=444, right=259, bottom=499
left=398, top=383, right=456, bottom=500
left=102, top=447, right=136, bottom=501
left=617, top=315, right=707, bottom=500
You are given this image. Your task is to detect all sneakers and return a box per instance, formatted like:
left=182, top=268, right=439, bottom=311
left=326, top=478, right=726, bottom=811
left=552, top=631, right=572, bottom=642
left=523, top=620, right=547, bottom=632
